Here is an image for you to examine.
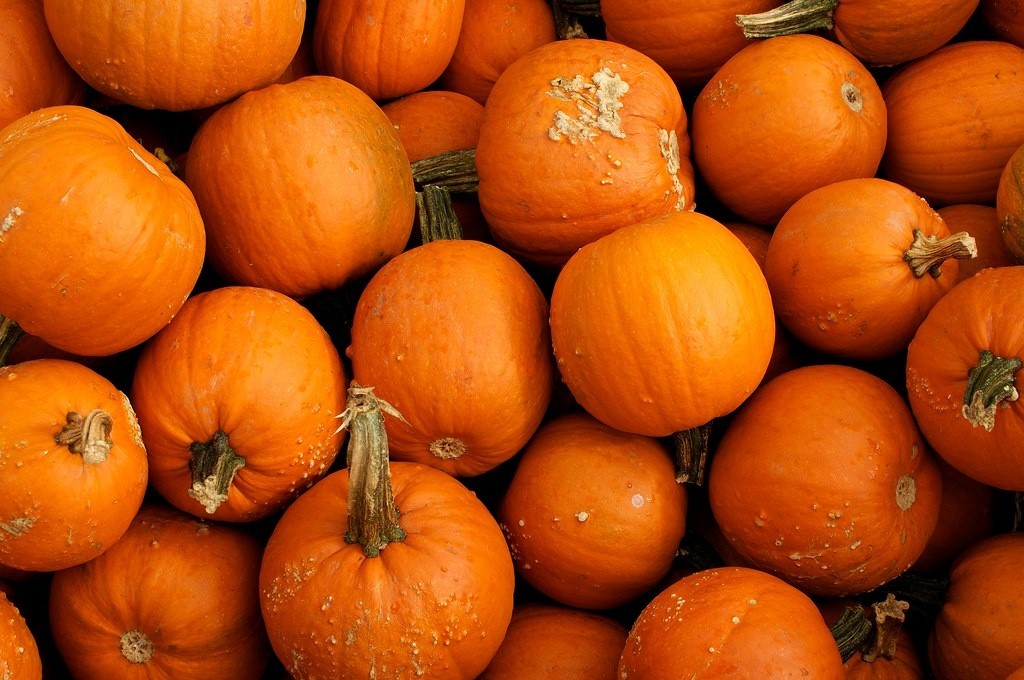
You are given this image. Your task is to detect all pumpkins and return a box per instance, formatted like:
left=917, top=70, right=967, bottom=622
left=0, top=0, right=1024, bottom=680
